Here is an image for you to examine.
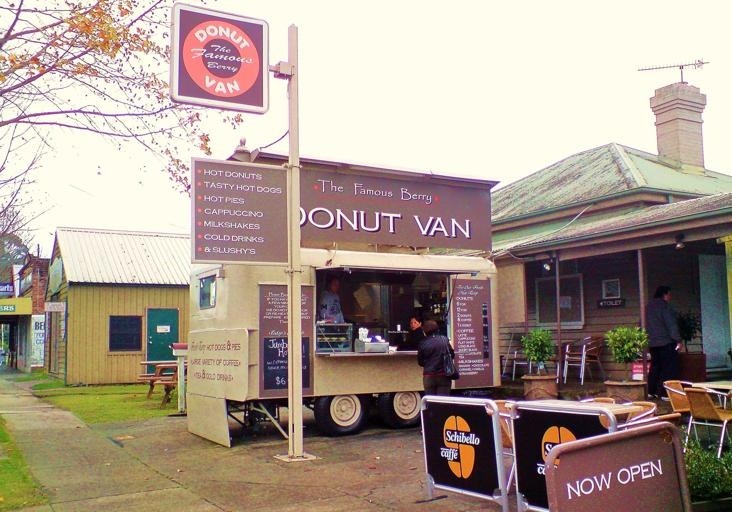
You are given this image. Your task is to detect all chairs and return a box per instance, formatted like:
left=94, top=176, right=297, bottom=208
left=489, top=397, right=681, bottom=499
left=500, top=333, right=607, bottom=387
left=662, top=379, right=732, bottom=459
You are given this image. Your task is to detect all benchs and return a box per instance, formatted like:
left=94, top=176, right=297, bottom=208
left=136, top=372, right=187, bottom=411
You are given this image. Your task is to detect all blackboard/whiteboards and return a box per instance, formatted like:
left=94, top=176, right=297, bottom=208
left=257, top=283, right=314, bottom=398
left=452, top=278, right=494, bottom=389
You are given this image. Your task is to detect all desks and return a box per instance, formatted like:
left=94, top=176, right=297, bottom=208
left=138, top=360, right=187, bottom=410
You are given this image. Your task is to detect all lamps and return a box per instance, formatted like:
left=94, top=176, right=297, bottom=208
left=542, top=258, right=554, bottom=271
left=674, top=233, right=685, bottom=250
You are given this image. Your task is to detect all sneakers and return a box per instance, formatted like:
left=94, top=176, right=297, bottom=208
left=647, top=394, right=670, bottom=401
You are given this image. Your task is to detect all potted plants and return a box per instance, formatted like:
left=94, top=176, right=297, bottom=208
left=521, top=326, right=558, bottom=400
left=605, top=324, right=651, bottom=419
left=675, top=309, right=706, bottom=382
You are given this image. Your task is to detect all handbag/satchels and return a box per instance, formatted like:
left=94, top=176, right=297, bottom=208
left=441, top=336, right=459, bottom=380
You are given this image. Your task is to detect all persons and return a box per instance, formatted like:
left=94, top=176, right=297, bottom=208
left=646, top=286, right=683, bottom=401
left=406, top=317, right=424, bottom=350
left=418, top=319, right=455, bottom=396
left=319, top=277, right=344, bottom=324
